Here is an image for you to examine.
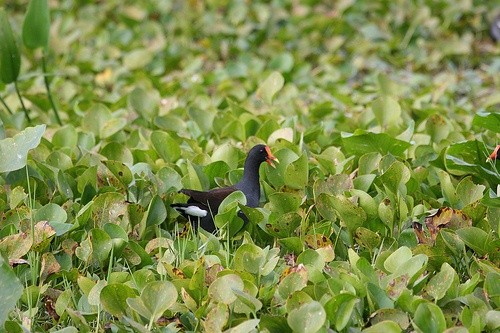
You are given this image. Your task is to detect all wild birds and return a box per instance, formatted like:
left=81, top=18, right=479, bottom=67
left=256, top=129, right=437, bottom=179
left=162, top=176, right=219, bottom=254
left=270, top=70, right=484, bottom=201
left=170, top=144, right=280, bottom=241
